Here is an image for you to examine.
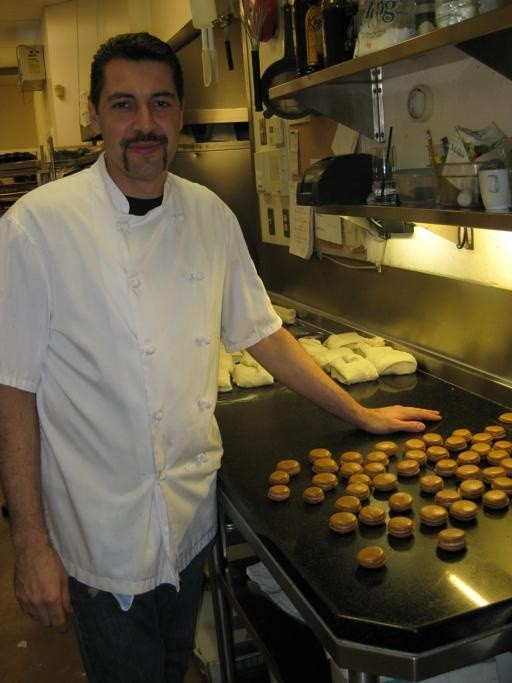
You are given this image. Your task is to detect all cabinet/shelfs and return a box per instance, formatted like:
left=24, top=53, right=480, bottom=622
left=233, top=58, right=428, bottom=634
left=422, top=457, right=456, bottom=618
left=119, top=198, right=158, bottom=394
left=168, top=109, right=262, bottom=253
left=269, top=4, right=512, bottom=233
left=0, top=137, right=99, bottom=218
left=213, top=371, right=511, bottom=682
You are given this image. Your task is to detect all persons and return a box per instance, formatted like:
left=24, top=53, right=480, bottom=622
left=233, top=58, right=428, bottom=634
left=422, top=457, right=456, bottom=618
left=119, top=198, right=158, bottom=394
left=0, top=33, right=443, bottom=683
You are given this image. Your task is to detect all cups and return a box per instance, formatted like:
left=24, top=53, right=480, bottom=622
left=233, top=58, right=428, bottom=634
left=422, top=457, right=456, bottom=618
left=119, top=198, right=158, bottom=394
left=368, top=143, right=397, bottom=204
left=477, top=168, right=512, bottom=209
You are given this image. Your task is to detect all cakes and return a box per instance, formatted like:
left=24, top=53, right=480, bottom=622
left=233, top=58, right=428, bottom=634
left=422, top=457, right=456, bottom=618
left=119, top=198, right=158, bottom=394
left=501, top=457, right=512, bottom=476
left=269, top=485, right=290, bottom=502
left=303, top=487, right=325, bottom=504
left=373, top=473, right=398, bottom=492
left=426, top=446, right=449, bottom=463
left=347, top=474, right=371, bottom=486
left=423, top=433, right=443, bottom=448
left=487, top=449, right=509, bottom=465
left=346, top=482, right=370, bottom=500
left=308, top=448, right=332, bottom=462
left=389, top=516, right=412, bottom=539
left=337, top=496, right=362, bottom=514
left=499, top=413, right=512, bottom=424
left=483, top=466, right=507, bottom=485
left=458, top=480, right=485, bottom=500
left=375, top=440, right=398, bottom=456
left=389, top=492, right=414, bottom=511
left=403, top=451, right=427, bottom=466
left=434, top=489, right=461, bottom=508
left=455, top=464, right=481, bottom=483
left=312, top=458, right=338, bottom=474
left=367, top=463, right=385, bottom=476
left=419, top=474, right=444, bottom=494
left=456, top=450, right=481, bottom=466
left=444, top=436, right=468, bottom=452
left=494, top=441, right=512, bottom=454
left=472, top=433, right=492, bottom=445
left=438, top=528, right=467, bottom=553
left=404, top=439, right=426, bottom=452
left=420, top=505, right=448, bottom=528
left=269, top=471, right=290, bottom=485
left=339, top=463, right=363, bottom=478
left=340, top=451, right=362, bottom=466
left=367, top=452, right=390, bottom=466
left=448, top=500, right=478, bottom=521
left=357, top=546, right=387, bottom=569
left=483, top=491, right=509, bottom=510
left=312, top=473, right=337, bottom=490
left=491, top=478, right=512, bottom=496
left=359, top=505, right=385, bottom=526
left=276, top=460, right=300, bottom=476
left=330, top=512, right=358, bottom=534
left=485, top=426, right=506, bottom=440
left=397, top=460, right=420, bottom=478
left=471, top=443, right=490, bottom=459
left=452, top=428, right=472, bottom=442
left=434, top=458, right=457, bottom=477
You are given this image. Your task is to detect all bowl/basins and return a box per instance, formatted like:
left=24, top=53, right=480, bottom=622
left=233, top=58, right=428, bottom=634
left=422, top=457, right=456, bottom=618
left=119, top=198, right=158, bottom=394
left=426, top=161, right=485, bottom=211
left=394, top=167, right=439, bottom=204
